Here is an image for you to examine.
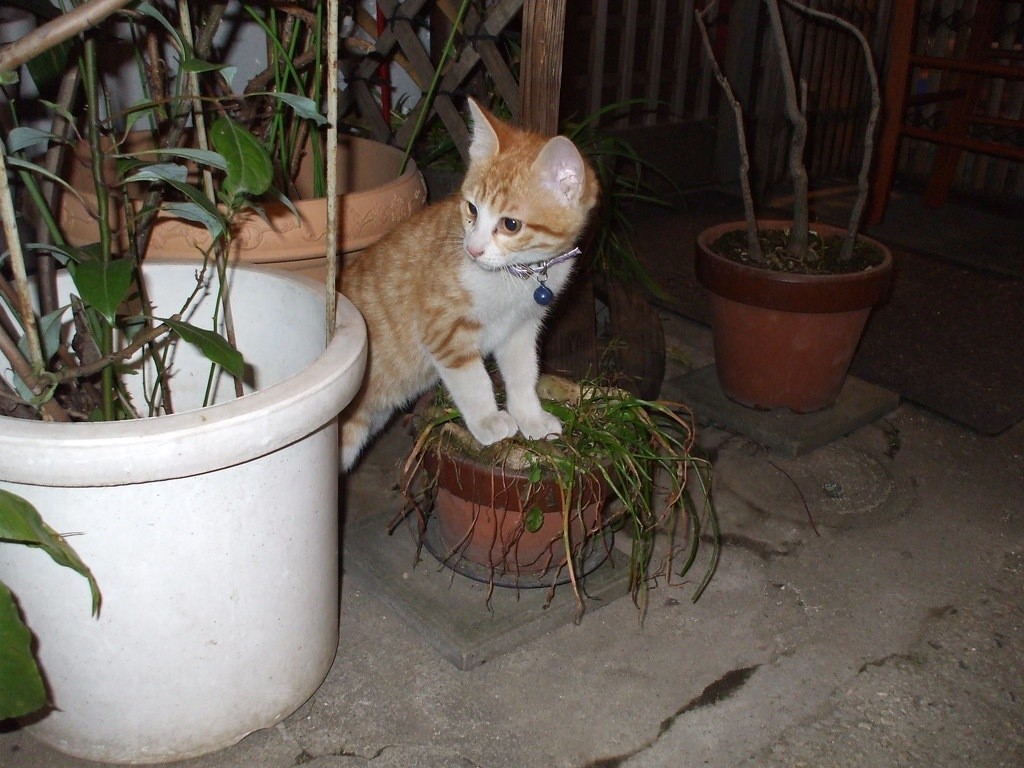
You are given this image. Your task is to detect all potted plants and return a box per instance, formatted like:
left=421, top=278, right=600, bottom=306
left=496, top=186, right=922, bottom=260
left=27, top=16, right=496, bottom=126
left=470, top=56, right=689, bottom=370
left=0, top=0, right=898, bottom=766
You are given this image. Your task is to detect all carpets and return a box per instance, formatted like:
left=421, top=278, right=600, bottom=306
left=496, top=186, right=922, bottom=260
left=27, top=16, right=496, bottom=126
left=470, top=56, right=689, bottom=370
left=589, top=183, right=1024, bottom=438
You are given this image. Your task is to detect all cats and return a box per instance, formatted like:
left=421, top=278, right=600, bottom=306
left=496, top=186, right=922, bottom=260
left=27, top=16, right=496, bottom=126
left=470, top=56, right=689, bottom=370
left=337, top=94, right=601, bottom=479
